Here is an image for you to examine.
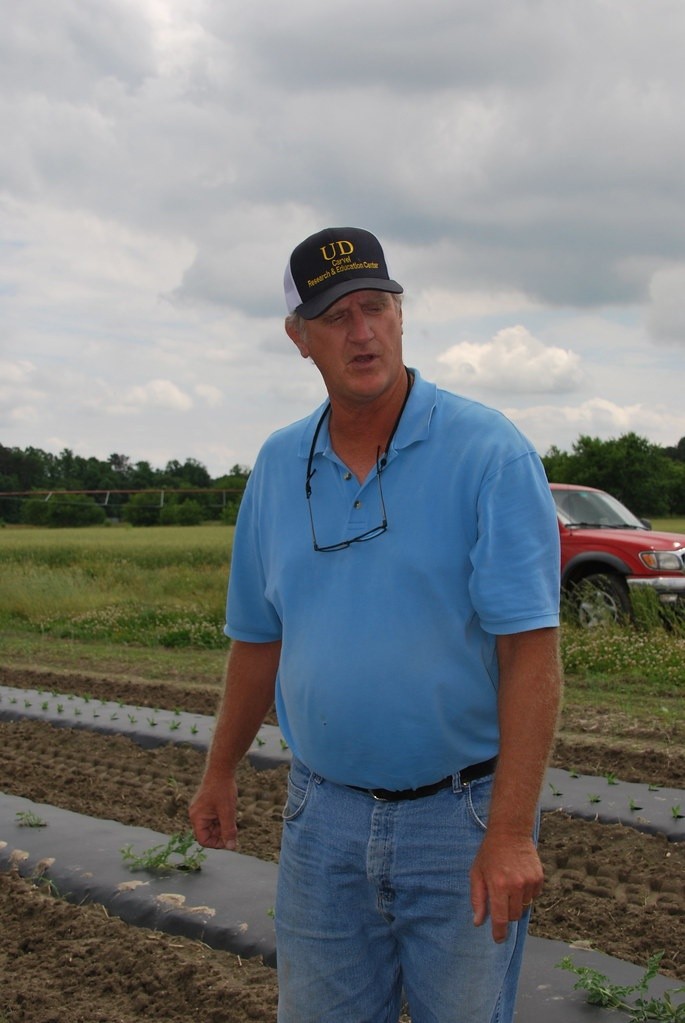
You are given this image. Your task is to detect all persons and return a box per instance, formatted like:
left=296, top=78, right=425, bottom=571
left=188, top=226, right=564, bottom=1023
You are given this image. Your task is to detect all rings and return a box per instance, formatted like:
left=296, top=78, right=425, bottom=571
left=523, top=899, right=533, bottom=906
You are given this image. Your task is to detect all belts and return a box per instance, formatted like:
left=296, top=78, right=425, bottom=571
left=344, top=756, right=497, bottom=804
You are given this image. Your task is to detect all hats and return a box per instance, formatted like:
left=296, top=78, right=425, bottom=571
left=282, top=227, right=403, bottom=320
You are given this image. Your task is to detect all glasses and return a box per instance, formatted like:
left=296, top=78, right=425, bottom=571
left=304, top=444, right=387, bottom=552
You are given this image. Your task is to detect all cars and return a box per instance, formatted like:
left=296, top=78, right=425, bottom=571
left=547, top=481, right=684, bottom=627
